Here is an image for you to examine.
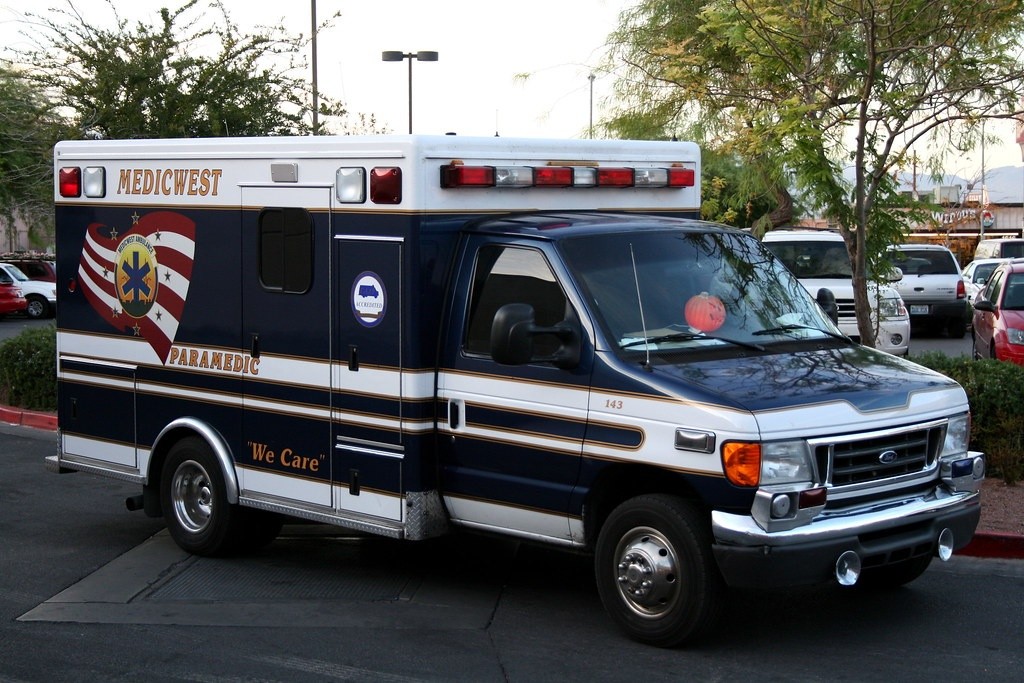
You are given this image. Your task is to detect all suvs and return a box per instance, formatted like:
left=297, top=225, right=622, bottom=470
left=737, top=225, right=911, bottom=358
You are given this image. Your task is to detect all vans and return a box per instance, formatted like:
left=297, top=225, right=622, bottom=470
left=972, top=238, right=1024, bottom=261
left=883, top=243, right=969, bottom=340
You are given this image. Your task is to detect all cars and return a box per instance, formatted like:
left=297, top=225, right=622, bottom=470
left=0, top=258, right=57, bottom=319
left=963, top=259, right=1024, bottom=367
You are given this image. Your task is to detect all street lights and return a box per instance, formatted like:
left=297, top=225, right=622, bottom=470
left=586, top=72, right=596, bottom=139
left=980, top=108, right=1000, bottom=240
left=381, top=49, right=439, bottom=133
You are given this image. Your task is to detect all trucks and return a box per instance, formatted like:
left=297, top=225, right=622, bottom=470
left=43, top=138, right=990, bottom=648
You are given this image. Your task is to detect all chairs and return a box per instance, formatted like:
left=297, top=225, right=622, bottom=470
left=900, top=264, right=907, bottom=273
left=817, top=247, right=852, bottom=274
left=1006, top=283, right=1024, bottom=307
left=975, top=271, right=990, bottom=282
left=648, top=277, right=687, bottom=327
left=917, top=265, right=940, bottom=274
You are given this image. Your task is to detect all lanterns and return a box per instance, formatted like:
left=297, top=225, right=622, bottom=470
left=685, top=291, right=726, bottom=333
